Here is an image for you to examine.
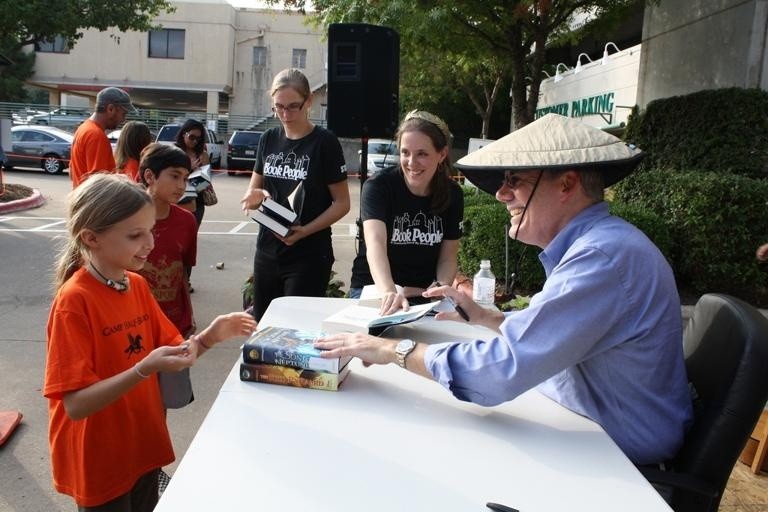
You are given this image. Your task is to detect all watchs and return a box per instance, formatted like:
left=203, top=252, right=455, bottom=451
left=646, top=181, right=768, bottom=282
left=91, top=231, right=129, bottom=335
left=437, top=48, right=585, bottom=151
left=395, top=338, right=416, bottom=370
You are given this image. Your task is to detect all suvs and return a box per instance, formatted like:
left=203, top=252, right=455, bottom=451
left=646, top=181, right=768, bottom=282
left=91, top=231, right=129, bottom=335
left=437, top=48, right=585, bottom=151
left=225, top=130, right=265, bottom=177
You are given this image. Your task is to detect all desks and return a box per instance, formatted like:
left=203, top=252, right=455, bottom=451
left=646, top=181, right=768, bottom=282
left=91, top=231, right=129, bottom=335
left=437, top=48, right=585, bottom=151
left=149, top=296, right=681, bottom=512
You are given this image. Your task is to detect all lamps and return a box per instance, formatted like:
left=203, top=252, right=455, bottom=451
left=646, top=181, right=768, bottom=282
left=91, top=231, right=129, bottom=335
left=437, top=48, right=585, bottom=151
left=552, top=41, right=623, bottom=85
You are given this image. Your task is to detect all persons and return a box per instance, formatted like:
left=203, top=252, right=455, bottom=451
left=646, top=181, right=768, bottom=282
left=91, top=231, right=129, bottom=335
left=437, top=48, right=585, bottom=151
left=136, top=141, right=197, bottom=498
left=40, top=167, right=258, bottom=511
left=174, top=118, right=218, bottom=293
left=114, top=119, right=150, bottom=184
left=241, top=67, right=351, bottom=320
left=313, top=112, right=693, bottom=503
left=348, top=109, right=465, bottom=315
left=68, top=87, right=136, bottom=190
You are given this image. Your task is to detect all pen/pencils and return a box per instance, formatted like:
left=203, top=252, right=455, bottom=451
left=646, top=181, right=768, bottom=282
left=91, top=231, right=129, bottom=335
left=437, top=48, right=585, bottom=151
left=435, top=280, right=470, bottom=322
left=487, top=502, right=520, bottom=512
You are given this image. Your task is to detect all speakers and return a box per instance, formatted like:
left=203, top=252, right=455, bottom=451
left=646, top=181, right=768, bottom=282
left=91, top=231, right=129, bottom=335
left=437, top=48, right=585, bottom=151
left=326, top=23, right=400, bottom=141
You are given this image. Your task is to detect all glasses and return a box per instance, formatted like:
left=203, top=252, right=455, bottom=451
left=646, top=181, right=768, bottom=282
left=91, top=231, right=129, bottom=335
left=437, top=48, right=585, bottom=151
left=271, top=96, right=310, bottom=114
left=186, top=133, right=204, bottom=143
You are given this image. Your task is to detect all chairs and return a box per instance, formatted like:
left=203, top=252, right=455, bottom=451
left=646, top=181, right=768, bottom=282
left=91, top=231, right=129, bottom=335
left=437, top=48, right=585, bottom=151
left=635, top=291, right=767, bottom=512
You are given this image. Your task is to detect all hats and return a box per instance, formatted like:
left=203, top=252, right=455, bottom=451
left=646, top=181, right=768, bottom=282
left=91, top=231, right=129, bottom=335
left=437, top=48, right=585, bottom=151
left=96, top=88, right=138, bottom=114
left=452, top=112, right=646, bottom=197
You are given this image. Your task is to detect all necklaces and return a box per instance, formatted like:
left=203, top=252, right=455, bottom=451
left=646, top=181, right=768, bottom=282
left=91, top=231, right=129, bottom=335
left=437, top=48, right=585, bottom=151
left=89, top=260, right=131, bottom=293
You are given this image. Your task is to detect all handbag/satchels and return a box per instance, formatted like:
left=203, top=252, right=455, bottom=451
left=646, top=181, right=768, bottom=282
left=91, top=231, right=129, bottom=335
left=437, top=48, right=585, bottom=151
left=202, top=186, right=218, bottom=206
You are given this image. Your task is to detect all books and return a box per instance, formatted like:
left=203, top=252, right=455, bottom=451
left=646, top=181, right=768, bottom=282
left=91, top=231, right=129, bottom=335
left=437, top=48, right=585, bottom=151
left=188, top=163, right=211, bottom=186
left=261, top=198, right=298, bottom=224
left=177, top=191, right=198, bottom=204
left=239, top=362, right=350, bottom=392
left=327, top=304, right=441, bottom=338
left=243, top=326, right=354, bottom=375
left=249, top=209, right=290, bottom=238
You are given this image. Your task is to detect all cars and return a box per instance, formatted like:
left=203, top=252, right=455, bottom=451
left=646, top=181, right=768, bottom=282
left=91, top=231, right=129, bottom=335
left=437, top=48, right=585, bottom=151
left=355, top=139, right=400, bottom=179
left=0, top=105, right=223, bottom=173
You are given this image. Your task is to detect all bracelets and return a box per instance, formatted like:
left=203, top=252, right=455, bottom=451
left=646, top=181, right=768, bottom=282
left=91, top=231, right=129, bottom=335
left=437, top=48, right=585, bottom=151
left=197, top=335, right=210, bottom=349
left=135, top=363, right=150, bottom=378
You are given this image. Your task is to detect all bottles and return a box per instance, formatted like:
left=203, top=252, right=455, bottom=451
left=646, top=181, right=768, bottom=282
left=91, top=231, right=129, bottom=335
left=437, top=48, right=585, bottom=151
left=472, top=260, right=496, bottom=314
